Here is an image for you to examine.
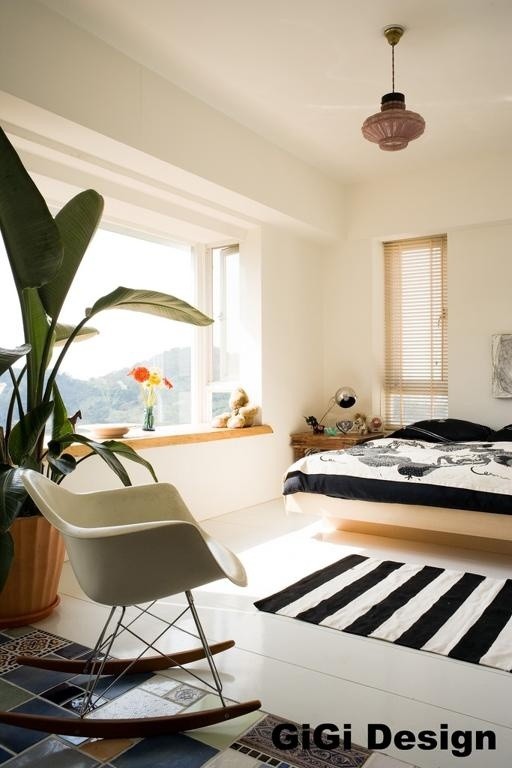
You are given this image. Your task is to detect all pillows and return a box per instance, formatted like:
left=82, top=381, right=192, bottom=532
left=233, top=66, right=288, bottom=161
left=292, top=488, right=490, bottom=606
left=386, top=418, right=495, bottom=443
left=495, top=423, right=511, bottom=441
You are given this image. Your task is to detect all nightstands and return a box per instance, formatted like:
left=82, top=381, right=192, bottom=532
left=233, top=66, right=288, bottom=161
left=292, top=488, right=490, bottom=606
left=291, top=433, right=386, bottom=469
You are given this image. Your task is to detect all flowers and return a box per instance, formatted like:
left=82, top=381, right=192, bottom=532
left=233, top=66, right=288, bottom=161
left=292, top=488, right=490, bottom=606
left=127, top=367, right=173, bottom=408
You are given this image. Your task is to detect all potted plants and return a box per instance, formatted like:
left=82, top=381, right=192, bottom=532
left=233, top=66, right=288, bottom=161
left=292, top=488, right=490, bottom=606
left=0, top=126, right=215, bottom=630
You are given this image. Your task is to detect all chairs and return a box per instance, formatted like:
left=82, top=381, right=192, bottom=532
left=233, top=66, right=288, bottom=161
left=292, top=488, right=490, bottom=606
left=0, top=469, right=262, bottom=740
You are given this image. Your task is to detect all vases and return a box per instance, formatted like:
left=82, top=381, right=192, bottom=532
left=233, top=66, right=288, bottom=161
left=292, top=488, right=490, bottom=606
left=144, top=407, right=158, bottom=432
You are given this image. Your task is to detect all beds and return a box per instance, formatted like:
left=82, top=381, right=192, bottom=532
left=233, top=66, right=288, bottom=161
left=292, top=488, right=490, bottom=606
left=281, top=418, right=512, bottom=555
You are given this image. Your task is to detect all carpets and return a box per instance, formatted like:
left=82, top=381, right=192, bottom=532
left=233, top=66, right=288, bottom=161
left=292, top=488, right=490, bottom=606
left=254, top=554, right=511, bottom=674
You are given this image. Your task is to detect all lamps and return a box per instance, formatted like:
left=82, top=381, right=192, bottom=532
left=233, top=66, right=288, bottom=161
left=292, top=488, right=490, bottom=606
left=361, top=26, right=427, bottom=152
left=309, top=387, right=358, bottom=434
